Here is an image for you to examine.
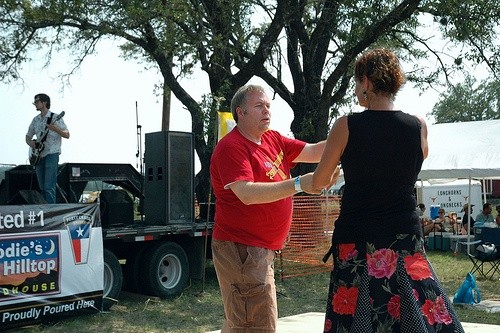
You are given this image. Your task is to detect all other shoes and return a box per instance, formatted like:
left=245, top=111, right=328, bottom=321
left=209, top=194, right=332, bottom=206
left=438, top=229, right=447, bottom=232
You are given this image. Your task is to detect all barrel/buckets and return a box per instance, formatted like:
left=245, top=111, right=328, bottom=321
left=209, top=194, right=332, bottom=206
left=430, top=205, right=441, bottom=220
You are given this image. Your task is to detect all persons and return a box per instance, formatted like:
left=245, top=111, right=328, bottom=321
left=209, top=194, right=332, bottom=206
left=312, top=48, right=466, bottom=333
left=210, top=85, right=327, bottom=333
left=26, top=93, right=70, bottom=204
left=417, top=202, right=500, bottom=257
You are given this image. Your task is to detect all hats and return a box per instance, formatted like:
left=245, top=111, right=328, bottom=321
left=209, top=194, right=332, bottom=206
left=461, top=204, right=475, bottom=212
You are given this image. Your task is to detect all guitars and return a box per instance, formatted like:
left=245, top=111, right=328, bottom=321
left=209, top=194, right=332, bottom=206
left=29, top=110, right=65, bottom=165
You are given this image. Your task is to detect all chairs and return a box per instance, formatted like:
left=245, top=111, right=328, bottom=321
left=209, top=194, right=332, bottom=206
left=468, top=225, right=500, bottom=280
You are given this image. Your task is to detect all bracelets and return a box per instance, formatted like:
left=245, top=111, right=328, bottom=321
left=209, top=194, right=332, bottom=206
left=294, top=175, right=304, bottom=193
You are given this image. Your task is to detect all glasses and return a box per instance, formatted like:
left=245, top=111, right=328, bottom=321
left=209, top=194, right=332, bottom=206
left=438, top=212, right=443, bottom=214
left=32, top=100, right=40, bottom=105
left=424, top=208, right=426, bottom=209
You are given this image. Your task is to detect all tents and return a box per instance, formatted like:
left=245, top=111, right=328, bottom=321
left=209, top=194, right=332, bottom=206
left=327, top=119, right=500, bottom=257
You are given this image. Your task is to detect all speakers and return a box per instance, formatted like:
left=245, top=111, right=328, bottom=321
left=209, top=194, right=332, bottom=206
left=145, top=131, right=194, bottom=226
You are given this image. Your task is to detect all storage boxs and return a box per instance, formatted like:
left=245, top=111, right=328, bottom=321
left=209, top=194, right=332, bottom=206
left=429, top=231, right=453, bottom=251
left=450, top=235, right=475, bottom=254
